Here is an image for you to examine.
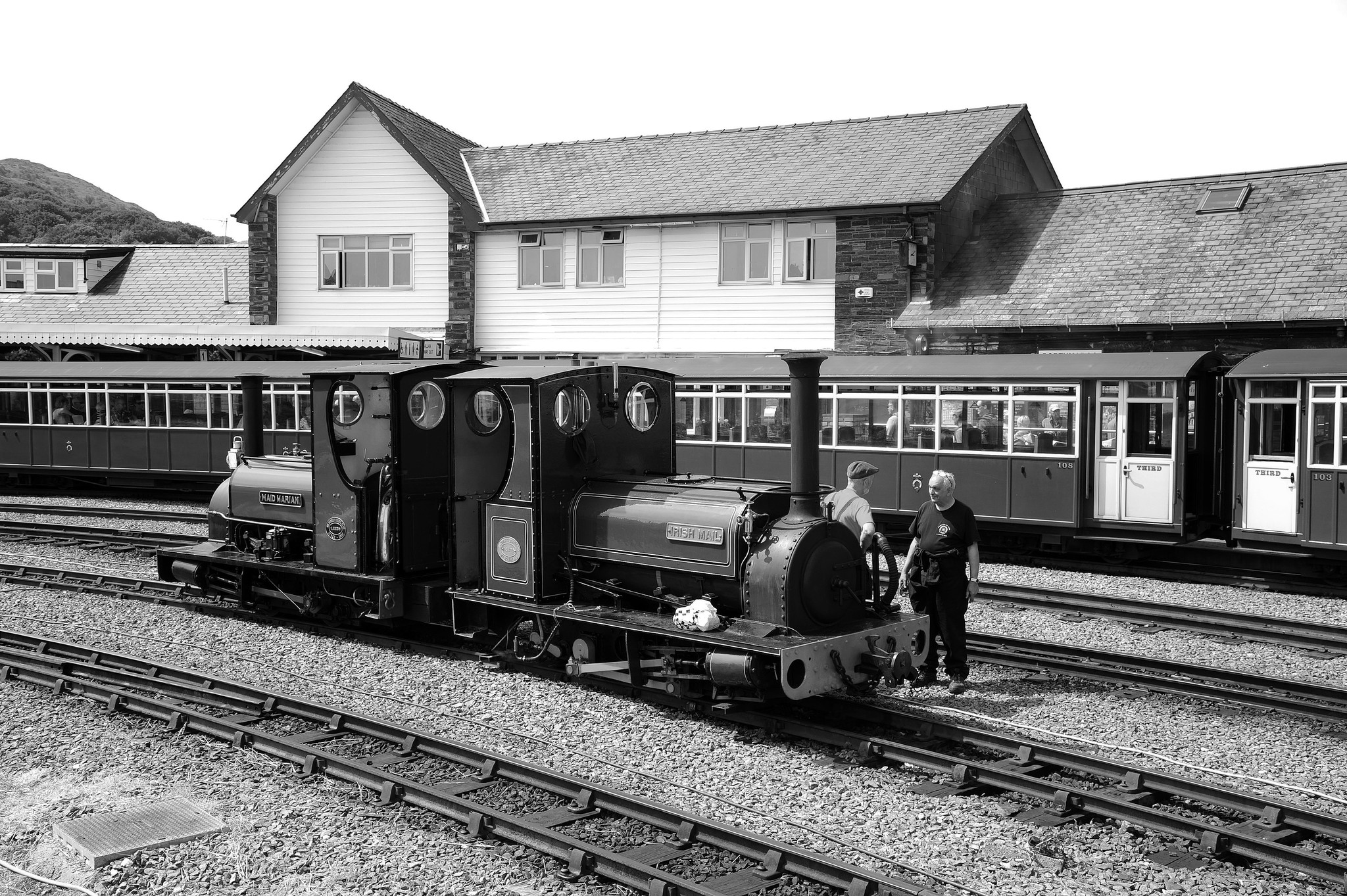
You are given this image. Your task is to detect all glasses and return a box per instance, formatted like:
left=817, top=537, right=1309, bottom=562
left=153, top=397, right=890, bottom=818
left=952, top=414, right=959, bottom=419
left=933, top=470, right=952, bottom=487
left=886, top=405, right=893, bottom=408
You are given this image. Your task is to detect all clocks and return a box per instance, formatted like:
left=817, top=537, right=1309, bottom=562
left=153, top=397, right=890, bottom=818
left=909, top=242, right=917, bottom=267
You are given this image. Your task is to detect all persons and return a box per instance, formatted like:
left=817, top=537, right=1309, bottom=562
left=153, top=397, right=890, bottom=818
left=820, top=461, right=879, bottom=600
left=898, top=468, right=979, bottom=694
left=53, top=394, right=311, bottom=431
left=952, top=400, right=1117, bottom=450
left=683, top=414, right=790, bottom=443
left=885, top=400, right=910, bottom=440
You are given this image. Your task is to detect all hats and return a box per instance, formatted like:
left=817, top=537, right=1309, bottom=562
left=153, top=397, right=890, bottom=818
left=847, top=461, right=879, bottom=479
left=1049, top=404, right=1061, bottom=411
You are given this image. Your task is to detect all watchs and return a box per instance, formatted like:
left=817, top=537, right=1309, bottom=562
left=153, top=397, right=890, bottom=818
left=970, top=577, right=979, bottom=584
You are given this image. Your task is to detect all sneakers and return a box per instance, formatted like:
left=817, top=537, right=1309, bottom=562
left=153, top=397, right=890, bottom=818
left=909, top=670, right=937, bottom=687
left=949, top=675, right=964, bottom=693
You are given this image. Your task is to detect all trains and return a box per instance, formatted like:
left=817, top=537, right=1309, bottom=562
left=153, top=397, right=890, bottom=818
left=0, top=339, right=1347, bottom=559
left=155, top=350, right=933, bottom=703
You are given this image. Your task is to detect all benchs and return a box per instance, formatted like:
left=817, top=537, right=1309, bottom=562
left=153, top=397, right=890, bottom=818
left=674, top=422, right=1054, bottom=456
left=41, top=412, right=296, bottom=429
